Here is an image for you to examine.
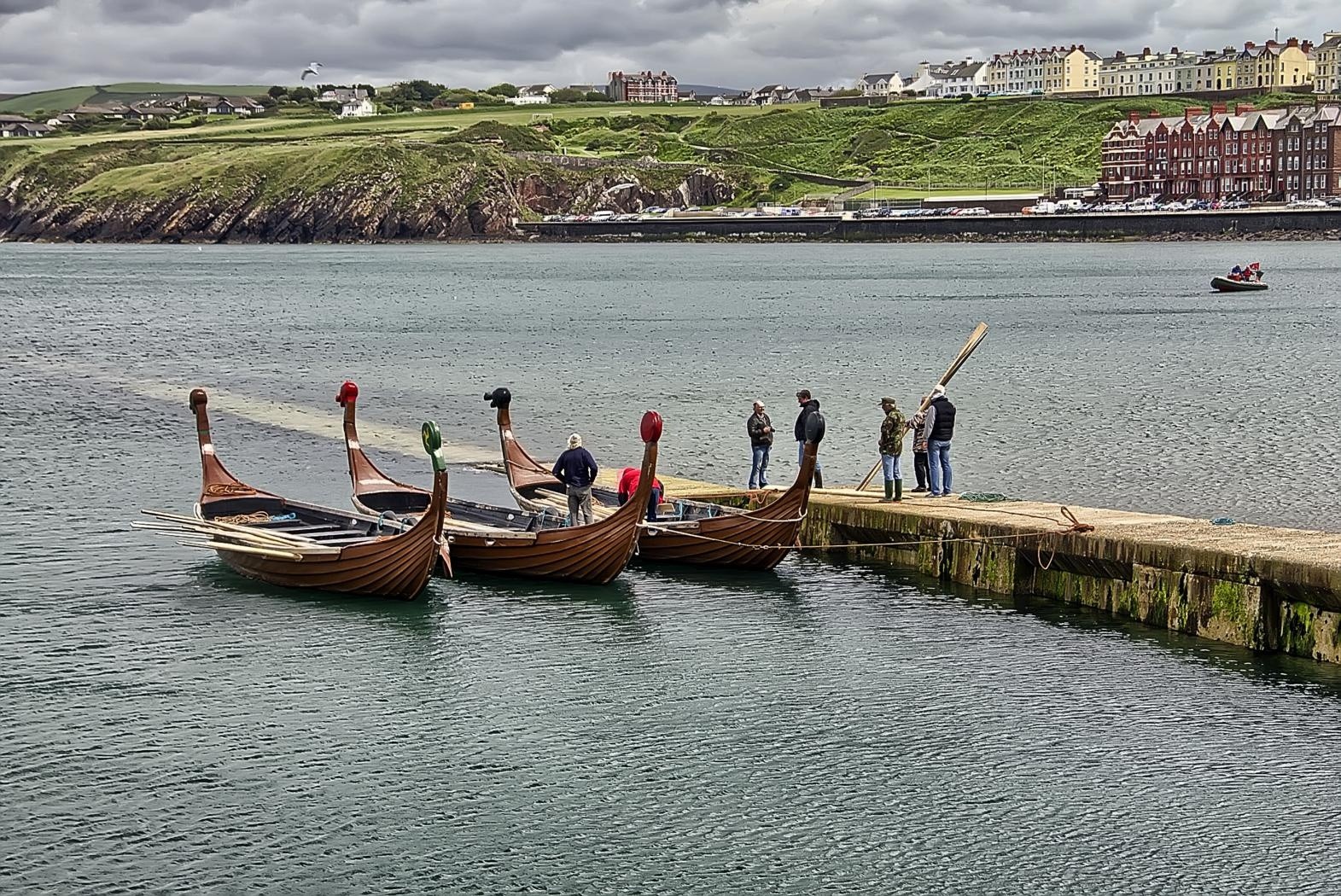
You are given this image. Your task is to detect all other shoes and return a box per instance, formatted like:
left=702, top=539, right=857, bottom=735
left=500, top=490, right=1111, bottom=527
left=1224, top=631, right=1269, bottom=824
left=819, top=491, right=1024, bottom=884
left=752, top=488, right=759, bottom=490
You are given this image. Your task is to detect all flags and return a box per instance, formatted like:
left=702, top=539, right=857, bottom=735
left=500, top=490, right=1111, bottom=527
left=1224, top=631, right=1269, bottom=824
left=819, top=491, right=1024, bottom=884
left=1249, top=263, right=1259, bottom=270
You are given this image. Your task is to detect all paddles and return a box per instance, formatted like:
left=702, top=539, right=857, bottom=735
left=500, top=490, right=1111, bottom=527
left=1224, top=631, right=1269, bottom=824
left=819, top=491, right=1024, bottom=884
left=761, top=484, right=914, bottom=500
left=130, top=508, right=321, bottom=546
left=855, top=321, right=989, bottom=494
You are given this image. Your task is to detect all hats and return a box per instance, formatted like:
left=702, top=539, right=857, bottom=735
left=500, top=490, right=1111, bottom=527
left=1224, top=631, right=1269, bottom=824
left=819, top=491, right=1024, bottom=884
left=878, top=398, right=896, bottom=405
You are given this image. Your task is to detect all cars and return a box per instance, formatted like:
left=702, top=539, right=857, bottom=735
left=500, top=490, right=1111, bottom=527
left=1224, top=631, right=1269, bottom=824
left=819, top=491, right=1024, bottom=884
left=541, top=191, right=1341, bottom=218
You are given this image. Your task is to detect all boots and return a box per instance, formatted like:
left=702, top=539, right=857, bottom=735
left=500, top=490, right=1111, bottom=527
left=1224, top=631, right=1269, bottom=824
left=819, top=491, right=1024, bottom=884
left=879, top=481, right=893, bottom=503
left=893, top=479, right=903, bottom=501
left=814, top=470, right=822, bottom=488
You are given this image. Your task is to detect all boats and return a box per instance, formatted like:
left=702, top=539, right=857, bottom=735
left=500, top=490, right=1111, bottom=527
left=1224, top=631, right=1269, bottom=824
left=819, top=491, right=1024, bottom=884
left=1210, top=275, right=1269, bottom=293
left=187, top=386, right=451, bottom=608
left=337, top=379, right=664, bottom=589
left=481, top=383, right=828, bottom=579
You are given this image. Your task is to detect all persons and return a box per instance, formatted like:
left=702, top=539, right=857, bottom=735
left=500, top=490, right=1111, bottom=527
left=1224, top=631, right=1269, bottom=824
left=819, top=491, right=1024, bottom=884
left=552, top=433, right=598, bottom=526
left=905, top=396, right=933, bottom=492
left=1232, top=265, right=1252, bottom=281
left=1126, top=204, right=1129, bottom=212
left=747, top=401, right=774, bottom=490
left=878, top=396, right=906, bottom=503
left=921, top=384, right=957, bottom=497
left=794, top=389, right=824, bottom=488
left=618, top=468, right=664, bottom=522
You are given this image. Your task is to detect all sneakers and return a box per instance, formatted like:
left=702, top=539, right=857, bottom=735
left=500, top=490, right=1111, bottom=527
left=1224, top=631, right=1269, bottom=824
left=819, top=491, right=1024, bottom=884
left=942, top=493, right=951, bottom=496
left=911, top=487, right=928, bottom=492
left=925, top=493, right=942, bottom=497
left=926, top=488, right=931, bottom=491
